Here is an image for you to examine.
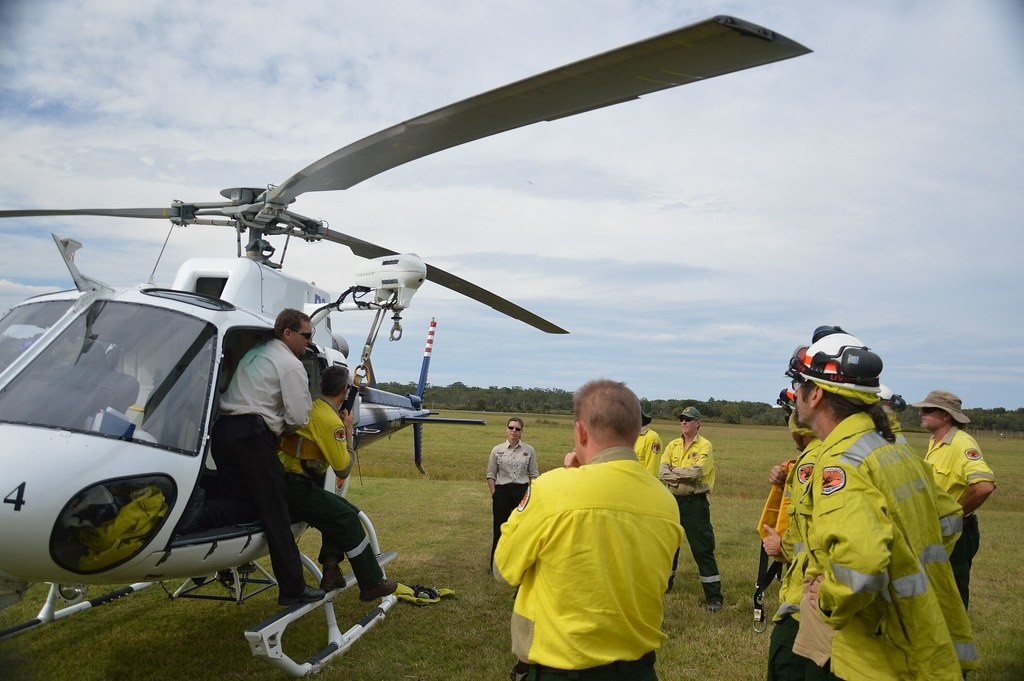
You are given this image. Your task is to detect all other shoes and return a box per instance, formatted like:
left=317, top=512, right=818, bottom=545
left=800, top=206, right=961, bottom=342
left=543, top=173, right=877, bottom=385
left=706, top=601, right=723, bottom=612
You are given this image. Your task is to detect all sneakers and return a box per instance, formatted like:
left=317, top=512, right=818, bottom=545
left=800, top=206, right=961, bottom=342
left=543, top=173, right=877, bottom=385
left=359, top=578, right=398, bottom=603
left=320, top=569, right=347, bottom=592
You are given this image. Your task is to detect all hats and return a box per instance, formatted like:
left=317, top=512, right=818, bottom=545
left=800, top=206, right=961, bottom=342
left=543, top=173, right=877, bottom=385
left=642, top=410, right=651, bottom=427
left=677, top=406, right=702, bottom=419
left=912, top=390, right=971, bottom=423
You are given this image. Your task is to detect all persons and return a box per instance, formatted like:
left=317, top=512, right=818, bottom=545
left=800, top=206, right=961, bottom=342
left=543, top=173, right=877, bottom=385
left=488, top=417, right=540, bottom=574
left=659, top=407, right=723, bottom=610
left=763, top=324, right=998, bottom=681
left=633, top=408, right=662, bottom=481
left=488, top=380, right=685, bottom=681
left=13, top=332, right=139, bottom=436
left=210, top=308, right=398, bottom=605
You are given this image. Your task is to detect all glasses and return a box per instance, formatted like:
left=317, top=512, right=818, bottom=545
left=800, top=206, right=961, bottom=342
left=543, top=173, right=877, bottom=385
left=291, top=329, right=311, bottom=340
left=508, top=426, right=521, bottom=431
left=789, top=345, right=809, bottom=369
left=791, top=379, right=814, bottom=390
left=922, top=407, right=944, bottom=414
left=679, top=417, right=698, bottom=422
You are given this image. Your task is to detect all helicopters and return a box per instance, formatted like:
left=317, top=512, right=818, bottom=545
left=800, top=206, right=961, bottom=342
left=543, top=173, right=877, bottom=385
left=0, top=0, right=814, bottom=681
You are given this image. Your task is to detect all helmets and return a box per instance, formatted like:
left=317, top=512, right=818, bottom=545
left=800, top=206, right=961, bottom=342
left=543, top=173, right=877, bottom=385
left=779, top=380, right=816, bottom=437
left=877, top=384, right=900, bottom=415
left=800, top=333, right=881, bottom=392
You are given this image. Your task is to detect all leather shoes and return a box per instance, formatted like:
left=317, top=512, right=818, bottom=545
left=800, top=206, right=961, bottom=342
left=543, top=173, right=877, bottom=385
left=278, top=584, right=325, bottom=606
